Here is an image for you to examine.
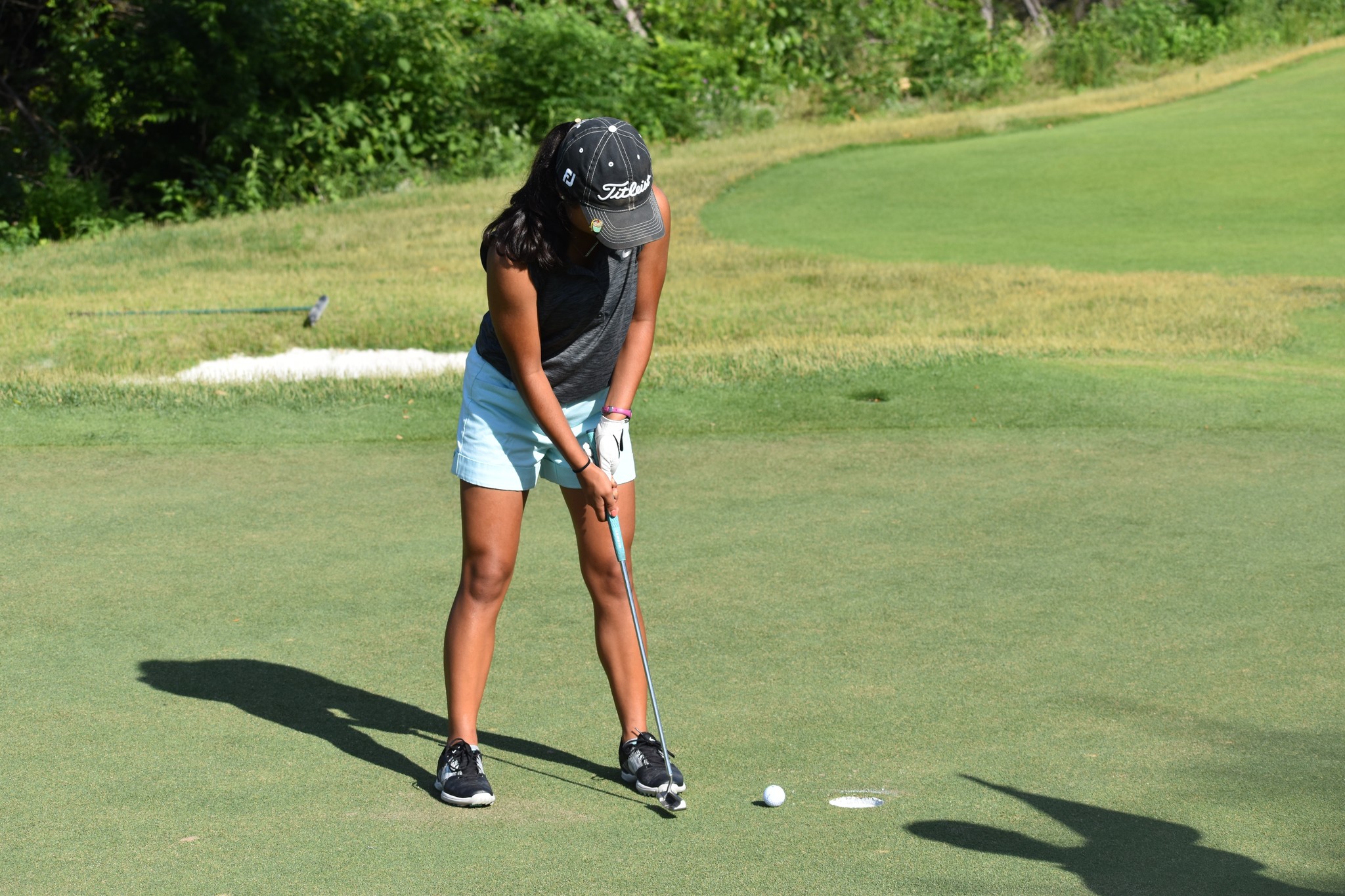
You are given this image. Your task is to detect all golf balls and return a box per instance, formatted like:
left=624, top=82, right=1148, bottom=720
left=764, top=785, right=785, bottom=807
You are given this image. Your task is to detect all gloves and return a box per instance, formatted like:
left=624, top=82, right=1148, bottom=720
left=595, top=414, right=630, bottom=482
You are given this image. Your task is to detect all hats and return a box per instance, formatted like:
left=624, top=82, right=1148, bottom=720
left=558, top=116, right=666, bottom=250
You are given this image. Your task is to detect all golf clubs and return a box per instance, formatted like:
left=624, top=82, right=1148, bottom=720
left=588, top=430, right=686, bottom=812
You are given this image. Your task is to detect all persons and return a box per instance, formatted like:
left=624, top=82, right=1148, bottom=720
left=430, top=117, right=685, bottom=807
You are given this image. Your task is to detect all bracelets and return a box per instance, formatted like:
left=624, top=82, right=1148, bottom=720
left=573, top=457, right=591, bottom=473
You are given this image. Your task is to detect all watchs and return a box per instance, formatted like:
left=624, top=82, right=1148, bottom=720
left=600, top=406, right=633, bottom=420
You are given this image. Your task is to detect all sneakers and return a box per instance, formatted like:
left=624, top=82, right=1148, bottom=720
left=433, top=737, right=495, bottom=806
left=619, top=728, right=686, bottom=796
